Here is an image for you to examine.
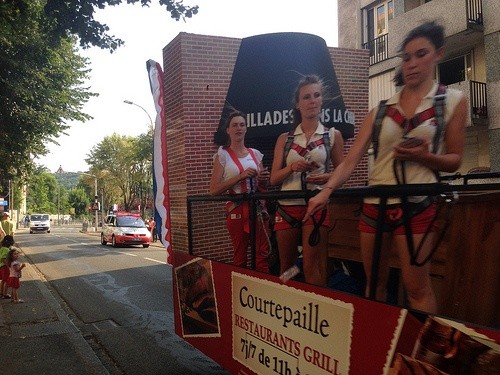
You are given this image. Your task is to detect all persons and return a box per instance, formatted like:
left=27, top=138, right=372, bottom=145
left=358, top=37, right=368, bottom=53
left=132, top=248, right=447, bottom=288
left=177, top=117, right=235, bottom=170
left=271, top=75, right=344, bottom=288
left=209, top=113, right=270, bottom=273
left=0, top=211, right=29, bottom=303
left=302, top=21, right=468, bottom=314
left=145, top=217, right=158, bottom=242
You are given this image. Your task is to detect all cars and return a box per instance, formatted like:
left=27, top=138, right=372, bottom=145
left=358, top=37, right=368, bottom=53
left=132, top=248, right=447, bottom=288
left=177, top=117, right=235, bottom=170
left=100, top=213, right=152, bottom=248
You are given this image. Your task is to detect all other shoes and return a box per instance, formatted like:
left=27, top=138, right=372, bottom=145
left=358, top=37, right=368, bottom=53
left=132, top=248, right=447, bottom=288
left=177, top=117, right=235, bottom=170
left=0, top=294, right=11, bottom=299
left=11, top=299, right=24, bottom=303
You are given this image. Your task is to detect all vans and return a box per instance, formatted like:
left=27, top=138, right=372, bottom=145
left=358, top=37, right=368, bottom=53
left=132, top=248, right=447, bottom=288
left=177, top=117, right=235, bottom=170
left=29, top=214, right=52, bottom=233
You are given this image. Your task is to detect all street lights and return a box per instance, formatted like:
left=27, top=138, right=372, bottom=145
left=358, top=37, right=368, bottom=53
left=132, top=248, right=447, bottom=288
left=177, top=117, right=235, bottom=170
left=123, top=99, right=153, bottom=135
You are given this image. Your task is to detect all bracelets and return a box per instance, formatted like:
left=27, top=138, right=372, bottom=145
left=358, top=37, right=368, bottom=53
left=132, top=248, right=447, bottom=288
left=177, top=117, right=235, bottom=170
left=322, top=186, right=336, bottom=191
